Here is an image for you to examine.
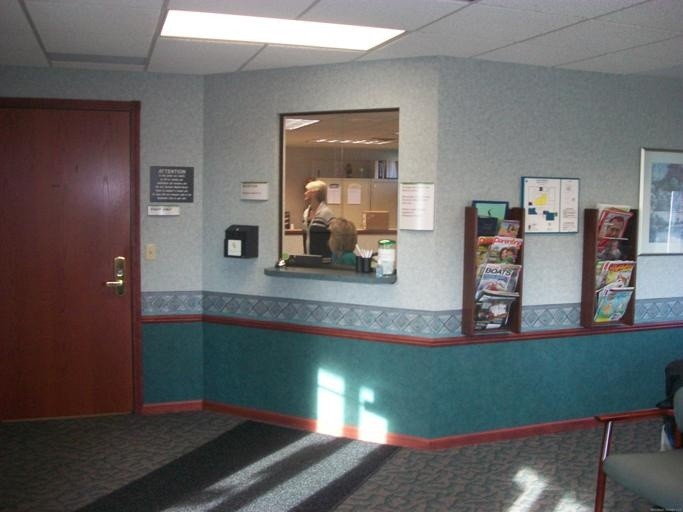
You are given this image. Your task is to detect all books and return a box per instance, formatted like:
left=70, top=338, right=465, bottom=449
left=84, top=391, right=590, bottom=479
left=475, top=219, right=524, bottom=330
left=594, top=203, right=635, bottom=326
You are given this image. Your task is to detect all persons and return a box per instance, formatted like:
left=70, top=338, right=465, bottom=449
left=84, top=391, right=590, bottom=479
left=327, top=217, right=358, bottom=265
left=301, top=180, right=336, bottom=264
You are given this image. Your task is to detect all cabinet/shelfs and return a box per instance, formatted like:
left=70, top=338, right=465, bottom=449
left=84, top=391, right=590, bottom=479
left=581, top=209, right=638, bottom=329
left=462, top=207, right=525, bottom=337
left=315, top=178, right=398, bottom=228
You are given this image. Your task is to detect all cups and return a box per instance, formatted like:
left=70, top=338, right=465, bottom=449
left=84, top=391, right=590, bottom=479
left=361, top=258, right=371, bottom=273
left=355, top=256, right=361, bottom=272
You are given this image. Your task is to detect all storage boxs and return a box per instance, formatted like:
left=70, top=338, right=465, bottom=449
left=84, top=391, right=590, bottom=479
left=362, top=211, right=389, bottom=231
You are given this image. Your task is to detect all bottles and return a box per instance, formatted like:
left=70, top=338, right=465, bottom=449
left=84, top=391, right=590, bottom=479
left=346, top=163, right=351, bottom=178
left=375, top=260, right=383, bottom=278
left=377, top=240, right=396, bottom=274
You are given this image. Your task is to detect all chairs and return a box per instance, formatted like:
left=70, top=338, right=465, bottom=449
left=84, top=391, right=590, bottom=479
left=595, top=360, right=683, bottom=512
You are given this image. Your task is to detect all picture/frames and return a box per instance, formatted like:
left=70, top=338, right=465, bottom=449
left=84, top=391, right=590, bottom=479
left=520, top=177, right=580, bottom=234
left=472, top=201, right=509, bottom=220
left=636, top=147, right=683, bottom=256
